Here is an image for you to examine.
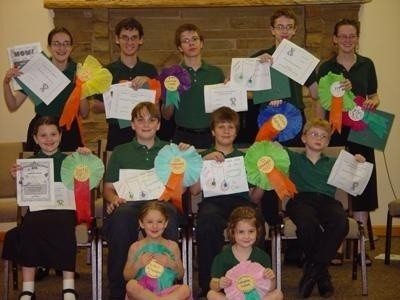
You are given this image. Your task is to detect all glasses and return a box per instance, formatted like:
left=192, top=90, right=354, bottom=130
left=337, top=35, right=357, bottom=39
left=181, top=37, right=199, bottom=43
left=274, top=24, right=292, bottom=30
left=120, top=35, right=138, bottom=41
left=52, top=41, right=71, bottom=47
left=308, top=132, right=326, bottom=139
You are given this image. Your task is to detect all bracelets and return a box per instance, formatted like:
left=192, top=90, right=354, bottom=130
left=112, top=195, right=118, bottom=200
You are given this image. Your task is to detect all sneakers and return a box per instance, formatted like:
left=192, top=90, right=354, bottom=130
left=20, top=290, right=35, bottom=300
left=62, top=289, right=78, bottom=300
left=316, top=268, right=333, bottom=298
left=298, top=263, right=317, bottom=298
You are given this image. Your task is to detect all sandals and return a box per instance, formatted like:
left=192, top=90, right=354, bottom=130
left=330, top=252, right=344, bottom=265
left=358, top=252, right=372, bottom=267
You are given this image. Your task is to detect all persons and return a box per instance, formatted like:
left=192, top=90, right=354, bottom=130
left=204, top=204, right=287, bottom=299
left=188, top=106, right=269, bottom=299
left=99, top=100, right=195, bottom=300
left=267, top=97, right=368, bottom=299
left=1, top=24, right=94, bottom=280
left=120, top=199, right=193, bottom=300
left=312, top=15, right=383, bottom=271
left=244, top=6, right=354, bottom=267
left=89, top=16, right=163, bottom=168
left=160, top=22, right=231, bottom=151
left=3, top=113, right=94, bottom=300
